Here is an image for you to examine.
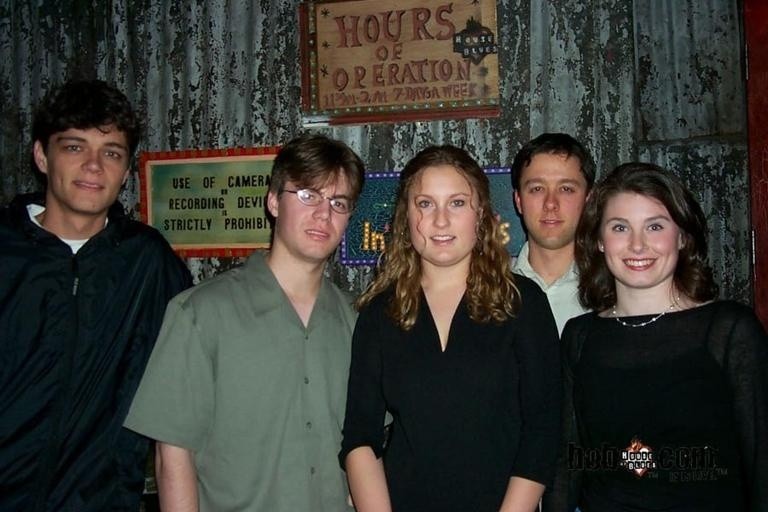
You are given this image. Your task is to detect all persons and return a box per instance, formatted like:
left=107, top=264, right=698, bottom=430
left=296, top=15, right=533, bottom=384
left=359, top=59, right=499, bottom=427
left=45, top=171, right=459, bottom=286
left=338, top=144, right=566, bottom=511
left=511, top=132, right=597, bottom=341
left=541, top=163, right=767, bottom=511
left=1, top=77, right=194, bottom=512
left=123, top=134, right=394, bottom=512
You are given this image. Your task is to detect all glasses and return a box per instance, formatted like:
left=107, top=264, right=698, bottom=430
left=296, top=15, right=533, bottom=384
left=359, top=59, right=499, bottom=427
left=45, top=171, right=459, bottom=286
left=281, top=188, right=355, bottom=214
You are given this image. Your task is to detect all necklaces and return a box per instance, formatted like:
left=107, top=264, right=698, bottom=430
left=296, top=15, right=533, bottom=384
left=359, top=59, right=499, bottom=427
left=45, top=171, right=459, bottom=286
left=612, top=292, right=682, bottom=327
